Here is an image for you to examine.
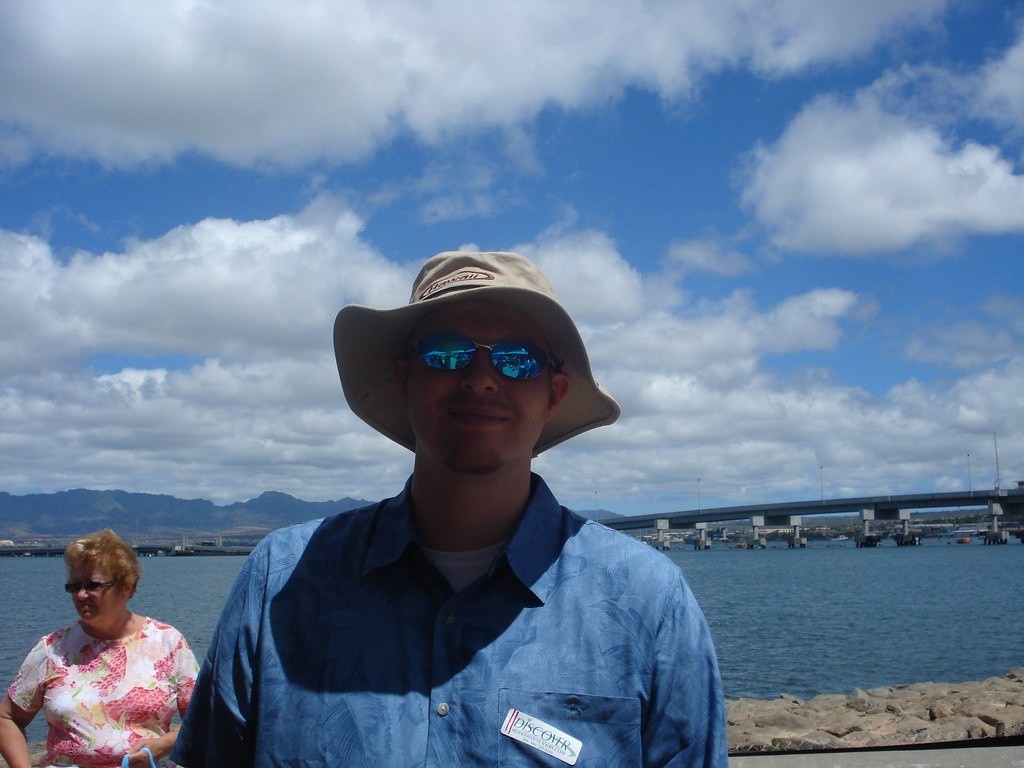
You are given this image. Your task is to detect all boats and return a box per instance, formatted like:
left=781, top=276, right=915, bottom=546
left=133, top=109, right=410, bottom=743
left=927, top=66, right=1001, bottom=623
left=955, top=538, right=971, bottom=544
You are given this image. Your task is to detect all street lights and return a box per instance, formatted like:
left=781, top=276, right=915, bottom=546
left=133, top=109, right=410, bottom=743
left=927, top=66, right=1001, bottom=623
left=698, top=478, right=701, bottom=511
left=820, top=466, right=824, bottom=501
left=967, top=453, right=972, bottom=492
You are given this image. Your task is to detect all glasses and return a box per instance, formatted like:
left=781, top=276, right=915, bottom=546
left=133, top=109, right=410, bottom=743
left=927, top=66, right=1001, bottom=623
left=406, top=331, right=564, bottom=381
left=65, top=578, right=119, bottom=593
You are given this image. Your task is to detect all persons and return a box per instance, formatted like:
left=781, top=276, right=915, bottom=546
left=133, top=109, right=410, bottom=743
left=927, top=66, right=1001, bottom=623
left=0, top=527, right=200, bottom=768
left=167, top=252, right=730, bottom=767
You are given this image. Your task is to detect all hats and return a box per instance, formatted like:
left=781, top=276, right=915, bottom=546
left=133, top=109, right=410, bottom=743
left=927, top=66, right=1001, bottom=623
left=332, top=251, right=622, bottom=459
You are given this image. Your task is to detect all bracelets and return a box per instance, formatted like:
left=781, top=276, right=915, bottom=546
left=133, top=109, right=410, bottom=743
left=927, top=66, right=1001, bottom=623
left=170, top=730, right=179, bottom=734
